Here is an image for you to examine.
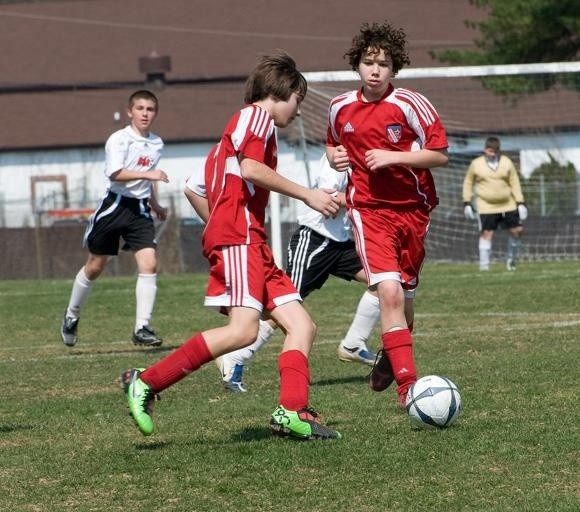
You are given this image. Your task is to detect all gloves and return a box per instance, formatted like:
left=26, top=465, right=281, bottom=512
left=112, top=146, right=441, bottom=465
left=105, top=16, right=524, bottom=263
left=463, top=204, right=474, bottom=221
left=517, top=204, right=528, bottom=220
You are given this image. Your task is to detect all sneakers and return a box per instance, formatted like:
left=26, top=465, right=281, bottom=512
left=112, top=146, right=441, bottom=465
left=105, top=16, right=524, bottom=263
left=269, top=406, right=342, bottom=442
left=337, top=342, right=380, bottom=365
left=223, top=367, right=248, bottom=393
left=507, top=258, right=515, bottom=272
left=479, top=262, right=490, bottom=272
left=131, top=325, right=163, bottom=347
left=121, top=368, right=154, bottom=436
left=370, top=348, right=395, bottom=392
left=61, top=310, right=80, bottom=347
left=396, top=376, right=415, bottom=406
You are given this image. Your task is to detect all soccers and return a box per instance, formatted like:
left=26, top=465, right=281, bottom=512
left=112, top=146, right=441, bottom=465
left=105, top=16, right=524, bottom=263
left=405, top=374, right=462, bottom=430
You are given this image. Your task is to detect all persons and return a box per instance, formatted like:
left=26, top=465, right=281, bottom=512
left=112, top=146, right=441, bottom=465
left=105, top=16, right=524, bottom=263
left=461, top=135, right=529, bottom=273
left=60, top=88, right=170, bottom=348
left=209, top=149, right=377, bottom=395
left=118, top=48, right=345, bottom=442
left=324, top=18, right=450, bottom=409
